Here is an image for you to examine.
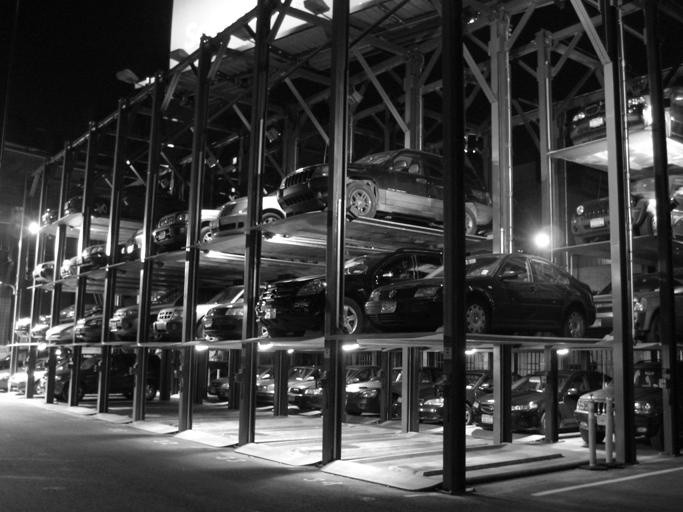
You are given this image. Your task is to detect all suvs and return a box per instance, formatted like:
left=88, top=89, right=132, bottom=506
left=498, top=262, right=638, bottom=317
left=572, top=358, right=683, bottom=446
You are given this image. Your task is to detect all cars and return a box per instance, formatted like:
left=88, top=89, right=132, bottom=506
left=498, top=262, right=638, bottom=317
left=11, top=248, right=455, bottom=340
left=568, top=84, right=673, bottom=146
left=569, top=174, right=683, bottom=238
left=391, top=368, right=526, bottom=425
left=590, top=270, right=682, bottom=342
left=1, top=145, right=494, bottom=259
left=469, top=368, right=611, bottom=437
left=361, top=252, right=597, bottom=337
left=0, top=348, right=414, bottom=417
